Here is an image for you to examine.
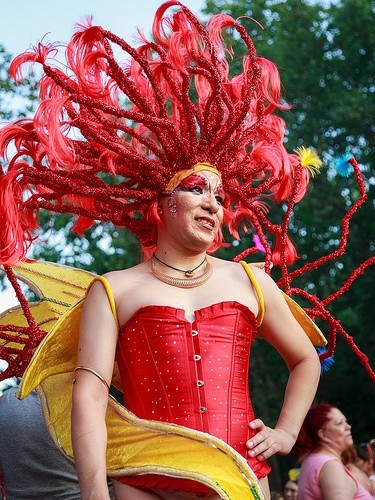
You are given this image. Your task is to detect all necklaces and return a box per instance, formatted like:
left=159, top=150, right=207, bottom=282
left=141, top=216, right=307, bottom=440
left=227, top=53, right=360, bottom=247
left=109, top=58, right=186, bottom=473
left=150, top=252, right=207, bottom=278
left=150, top=254, right=213, bottom=290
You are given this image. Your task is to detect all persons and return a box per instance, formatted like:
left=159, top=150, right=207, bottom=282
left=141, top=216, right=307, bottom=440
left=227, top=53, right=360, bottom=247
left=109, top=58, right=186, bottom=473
left=0, top=381, right=115, bottom=500
left=63, top=151, right=323, bottom=499
left=292, top=403, right=375, bottom=500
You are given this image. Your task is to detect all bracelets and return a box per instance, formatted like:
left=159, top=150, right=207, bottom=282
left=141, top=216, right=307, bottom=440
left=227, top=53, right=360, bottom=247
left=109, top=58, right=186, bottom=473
left=69, top=366, right=111, bottom=392
